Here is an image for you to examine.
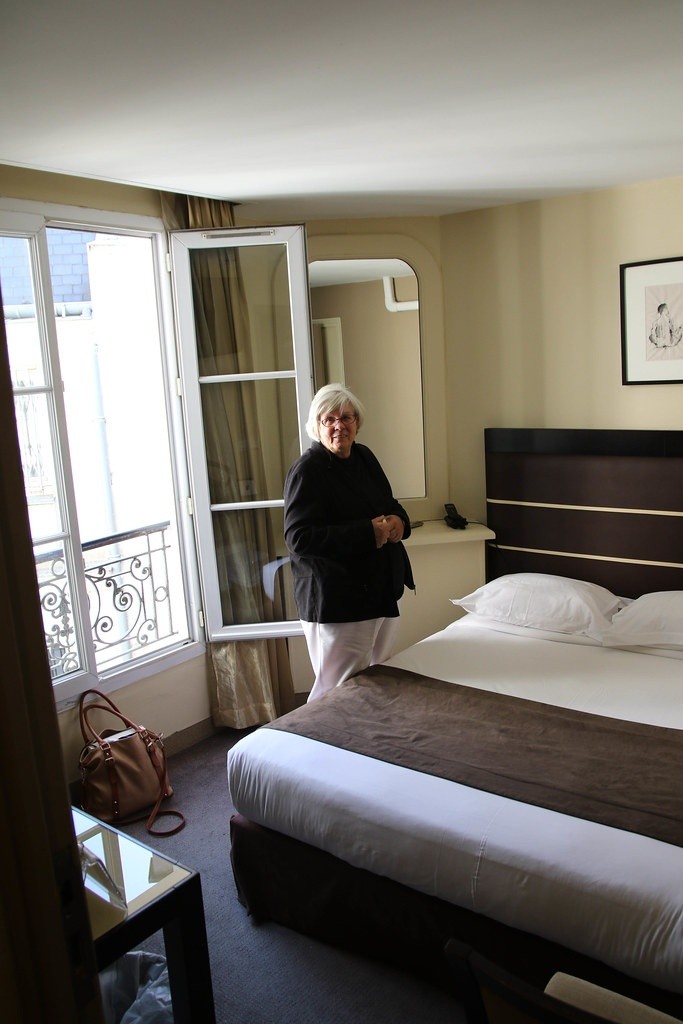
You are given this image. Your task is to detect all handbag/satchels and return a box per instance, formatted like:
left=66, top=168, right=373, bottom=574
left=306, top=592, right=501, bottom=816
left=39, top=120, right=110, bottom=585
left=75, top=689, right=174, bottom=824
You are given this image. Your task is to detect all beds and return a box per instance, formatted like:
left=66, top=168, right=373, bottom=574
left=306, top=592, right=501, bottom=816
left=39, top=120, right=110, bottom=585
left=227, top=423, right=683, bottom=1004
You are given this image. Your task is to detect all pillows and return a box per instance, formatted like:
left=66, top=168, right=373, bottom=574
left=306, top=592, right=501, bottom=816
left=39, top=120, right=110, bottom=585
left=609, top=590, right=683, bottom=648
left=449, top=572, right=622, bottom=643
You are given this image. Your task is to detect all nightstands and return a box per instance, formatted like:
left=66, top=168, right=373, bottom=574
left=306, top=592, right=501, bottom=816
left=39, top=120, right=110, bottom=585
left=391, top=518, right=497, bottom=660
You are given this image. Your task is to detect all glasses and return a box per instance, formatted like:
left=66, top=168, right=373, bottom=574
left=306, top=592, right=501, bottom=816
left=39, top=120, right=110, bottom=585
left=319, top=415, right=357, bottom=428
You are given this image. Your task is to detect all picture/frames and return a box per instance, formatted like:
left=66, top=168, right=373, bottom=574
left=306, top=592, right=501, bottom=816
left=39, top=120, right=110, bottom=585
left=619, top=256, right=683, bottom=386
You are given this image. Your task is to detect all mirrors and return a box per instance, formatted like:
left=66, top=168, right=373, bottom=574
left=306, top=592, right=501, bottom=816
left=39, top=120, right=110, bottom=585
left=305, top=258, right=427, bottom=500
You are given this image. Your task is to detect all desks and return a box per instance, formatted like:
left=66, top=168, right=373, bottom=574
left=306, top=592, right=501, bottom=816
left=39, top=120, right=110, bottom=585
left=69, top=803, right=216, bottom=1024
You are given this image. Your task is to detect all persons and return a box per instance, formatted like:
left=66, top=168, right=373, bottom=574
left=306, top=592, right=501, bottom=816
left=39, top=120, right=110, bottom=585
left=283, top=381, right=415, bottom=705
